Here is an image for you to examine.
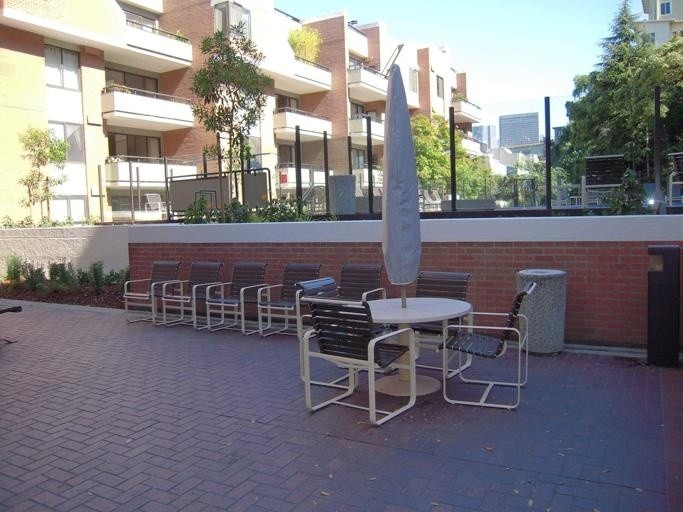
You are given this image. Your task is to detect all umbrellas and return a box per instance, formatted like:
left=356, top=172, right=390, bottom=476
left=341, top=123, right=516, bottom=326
left=342, top=63, right=472, bottom=398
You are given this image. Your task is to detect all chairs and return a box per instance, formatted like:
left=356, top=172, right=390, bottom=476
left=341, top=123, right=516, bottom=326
left=121, top=260, right=184, bottom=325
left=257, top=262, right=322, bottom=338
left=298, top=297, right=415, bottom=430
left=205, top=261, right=271, bottom=335
left=161, top=261, right=223, bottom=329
left=294, top=263, right=382, bottom=338
left=438, top=280, right=539, bottom=412
left=665, top=152, right=683, bottom=208
left=408, top=270, right=472, bottom=377
left=294, top=277, right=384, bottom=393
left=578, top=154, right=626, bottom=207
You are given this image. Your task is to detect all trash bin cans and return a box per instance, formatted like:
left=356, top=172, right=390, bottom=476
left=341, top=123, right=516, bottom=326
left=648, top=245, right=683, bottom=368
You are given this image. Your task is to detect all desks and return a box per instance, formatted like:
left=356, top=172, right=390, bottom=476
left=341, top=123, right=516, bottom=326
left=538, top=183, right=578, bottom=206
left=357, top=297, right=470, bottom=396
